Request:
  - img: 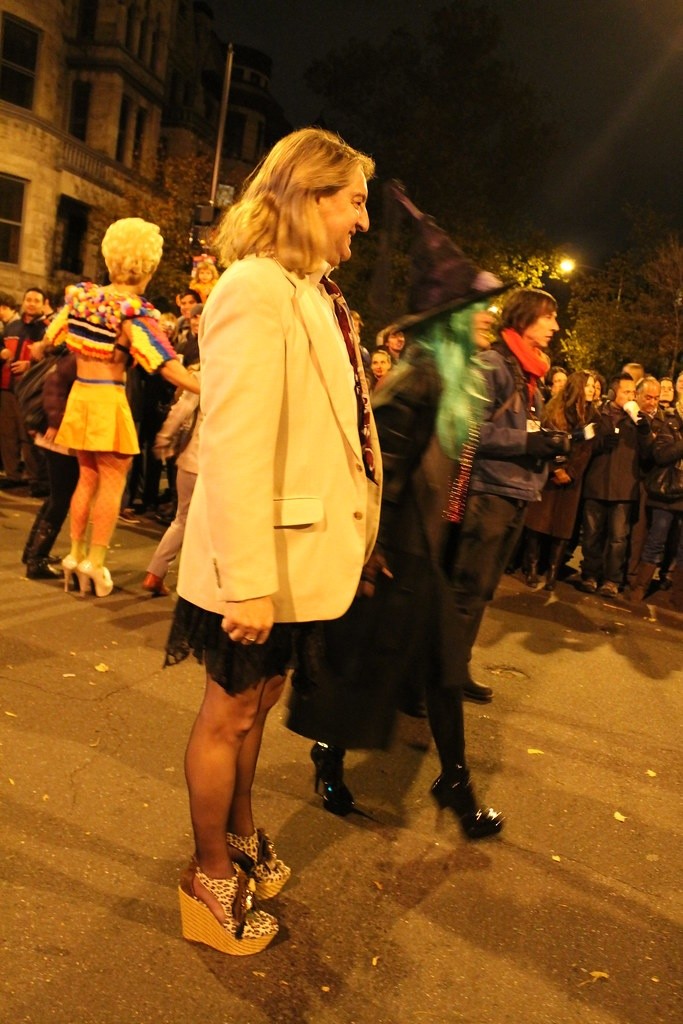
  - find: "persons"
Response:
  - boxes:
[449,288,560,698]
[27,217,201,597]
[21,355,79,580]
[192,262,217,295]
[0,287,65,497]
[505,363,683,608]
[142,370,204,595]
[283,300,502,840]
[120,289,205,525]
[160,129,383,956]
[350,310,405,390]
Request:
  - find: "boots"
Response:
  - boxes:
[624,562,656,601]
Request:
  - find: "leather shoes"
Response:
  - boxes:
[21,549,61,564]
[27,560,63,580]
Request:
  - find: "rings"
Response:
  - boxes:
[244,636,255,643]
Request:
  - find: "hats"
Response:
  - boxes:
[367,180,517,335]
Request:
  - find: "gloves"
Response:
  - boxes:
[525,431,567,461]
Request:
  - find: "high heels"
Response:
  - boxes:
[224,827,291,901]
[311,741,354,816]
[177,867,278,955]
[76,560,113,597]
[431,772,503,839]
[61,554,79,592]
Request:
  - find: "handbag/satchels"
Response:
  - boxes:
[643,428,683,501]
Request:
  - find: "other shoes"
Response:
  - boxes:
[397,695,426,716]
[463,676,493,702]
[142,573,170,595]
[118,508,141,523]
[29,485,48,497]
[507,555,620,597]
